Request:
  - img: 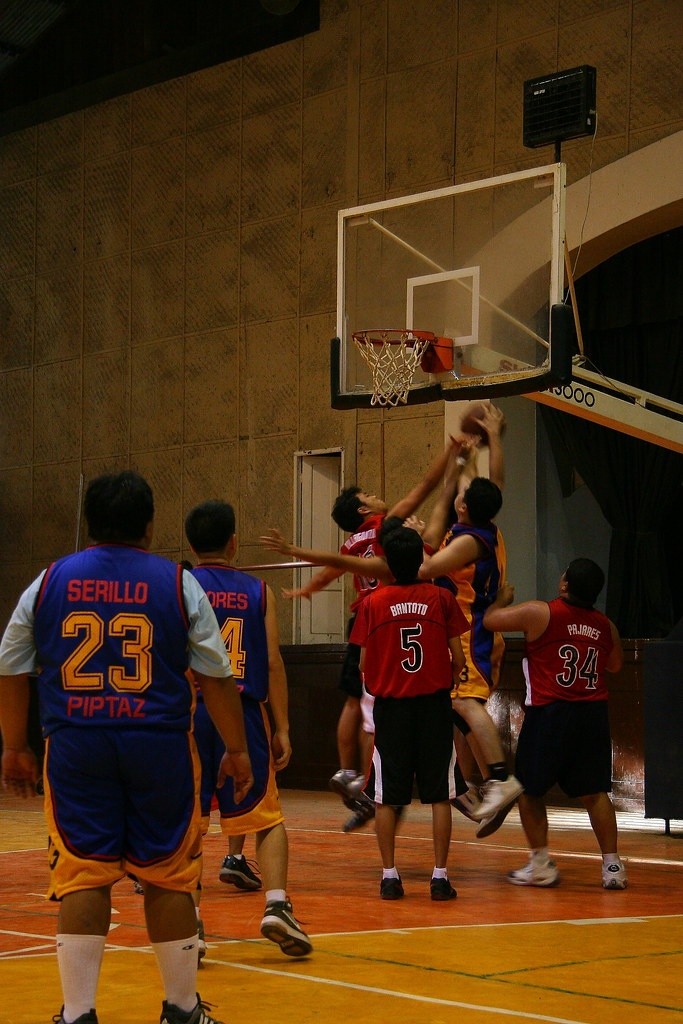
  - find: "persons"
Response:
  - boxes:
[348,527,472,901]
[260,402,526,839]
[148,501,313,963]
[0,471,254,1023]
[483,558,629,889]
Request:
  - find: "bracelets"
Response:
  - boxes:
[456,456,467,468]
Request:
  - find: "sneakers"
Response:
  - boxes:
[346,774,371,801]
[134,881,145,895]
[473,774,525,819]
[260,896,313,956]
[52,1004,98,1024]
[380,873,404,900]
[159,992,224,1024]
[430,878,456,900]
[474,799,516,838]
[219,854,262,889]
[342,806,375,833]
[197,919,206,964]
[329,768,358,802]
[505,860,561,888]
[601,856,629,888]
[450,780,483,824]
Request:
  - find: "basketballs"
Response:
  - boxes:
[461,401,506,440]
